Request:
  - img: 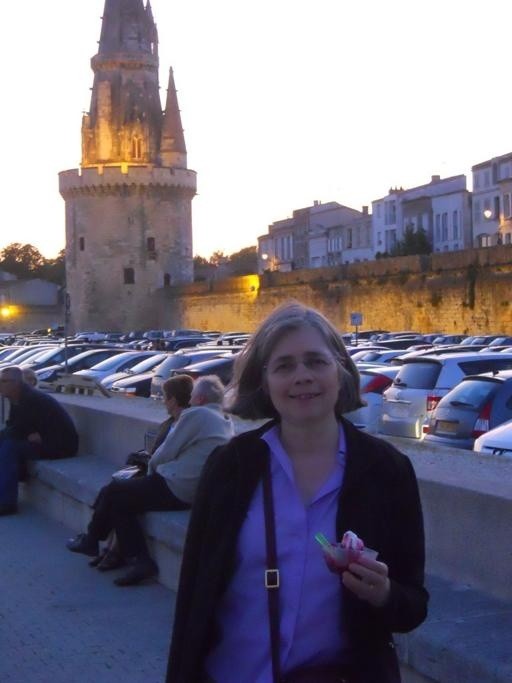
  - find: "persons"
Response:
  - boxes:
[89,374,195,571]
[0,367,79,515]
[66,374,235,586]
[165,302,430,683]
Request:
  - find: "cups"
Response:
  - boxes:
[327,544,378,574]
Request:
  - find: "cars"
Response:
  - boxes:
[0,327,252,399]
[341,329,512,457]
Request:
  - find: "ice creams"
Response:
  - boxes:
[318,531,365,573]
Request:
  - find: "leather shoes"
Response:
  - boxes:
[64,528,160,587]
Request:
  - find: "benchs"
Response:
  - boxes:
[19,450,509,681]
